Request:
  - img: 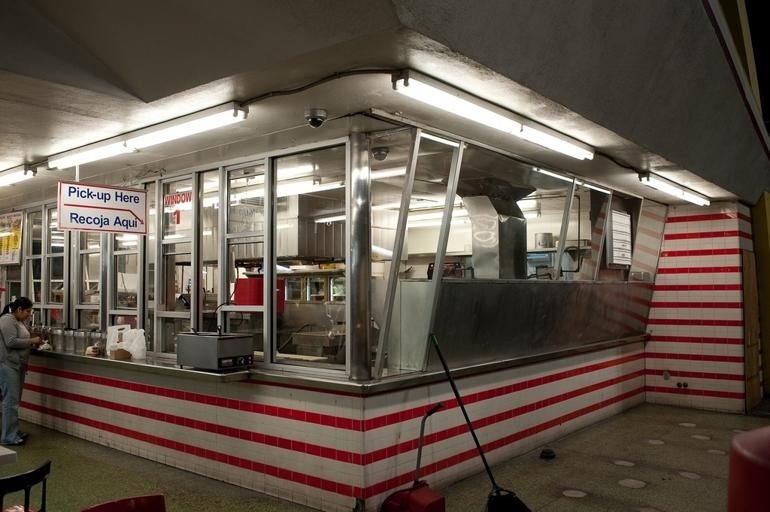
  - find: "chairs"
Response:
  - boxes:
[81,494,167,512]
[0,457,52,512]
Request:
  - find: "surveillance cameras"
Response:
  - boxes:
[304,109,327,128]
[372,147,389,160]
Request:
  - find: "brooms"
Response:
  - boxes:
[430,332,531,511]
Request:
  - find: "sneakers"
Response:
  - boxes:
[0,430,29,445]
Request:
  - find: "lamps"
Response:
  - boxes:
[391,68,595,162]
[637,170,710,207]
[44,101,249,170]
[2,166,37,186]
[166,157,410,206]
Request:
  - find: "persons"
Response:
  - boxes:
[0,296,45,446]
